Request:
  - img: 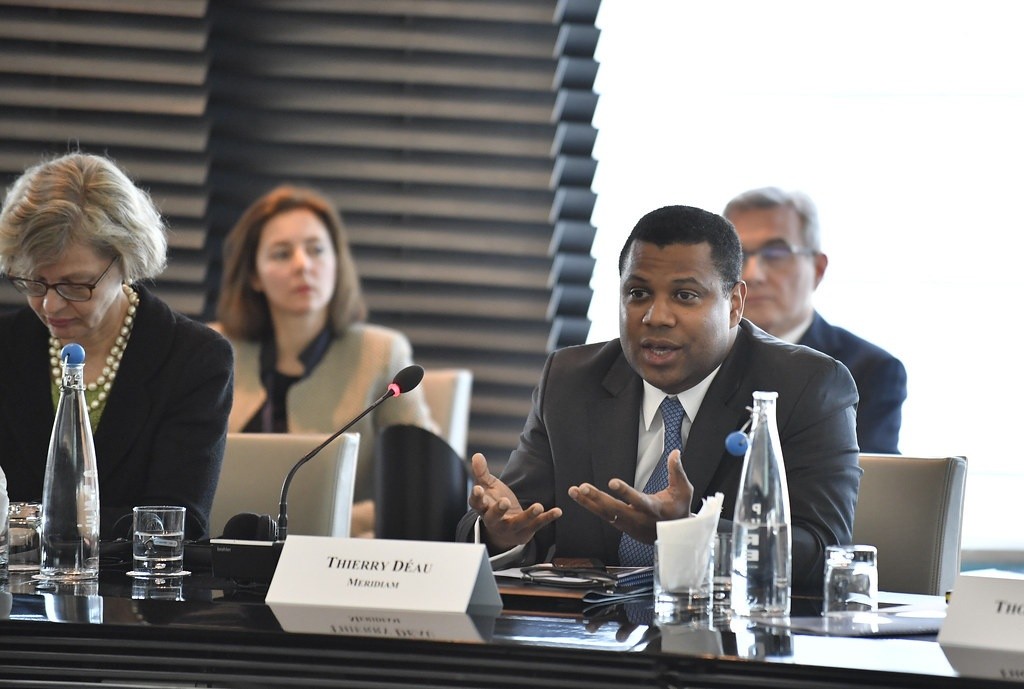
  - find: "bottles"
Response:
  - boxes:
[1,466,10,567]
[40,344,101,579]
[730,390,792,617]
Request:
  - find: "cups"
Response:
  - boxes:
[8,500,41,573]
[711,532,749,607]
[651,538,715,613]
[132,506,186,574]
[822,545,879,626]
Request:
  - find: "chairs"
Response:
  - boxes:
[852,453,968,597]
[208,432,361,540]
[420,367,473,460]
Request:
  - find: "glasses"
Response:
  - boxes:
[740,244,815,269]
[1,256,120,302]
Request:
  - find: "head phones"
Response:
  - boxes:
[192,513,278,546]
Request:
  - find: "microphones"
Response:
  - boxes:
[209,366,425,583]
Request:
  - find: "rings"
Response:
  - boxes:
[608,514,617,524]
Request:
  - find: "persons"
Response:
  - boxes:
[454,206,864,593]
[0,153,236,542]
[200,186,441,538]
[721,187,907,455]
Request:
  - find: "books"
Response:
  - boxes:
[491,561,655,588]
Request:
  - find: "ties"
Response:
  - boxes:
[618,399,686,568]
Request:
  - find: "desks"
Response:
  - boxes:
[0,538,1024,689]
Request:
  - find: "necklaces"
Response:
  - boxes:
[48,284,140,413]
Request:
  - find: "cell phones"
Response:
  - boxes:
[553,556,607,576]
